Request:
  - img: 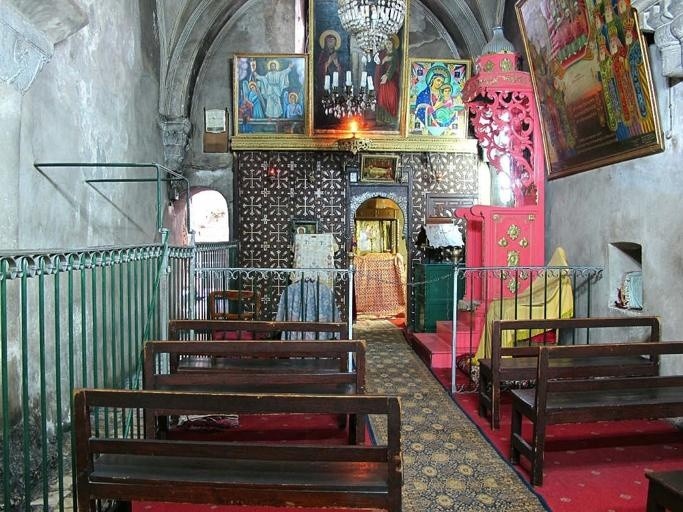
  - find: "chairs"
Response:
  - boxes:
[208,289,263,340]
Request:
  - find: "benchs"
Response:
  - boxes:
[477,316,683,488]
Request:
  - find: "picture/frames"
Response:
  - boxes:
[360,153,401,185]
[353,216,397,257]
[309,0,410,139]
[232,53,309,139]
[408,57,472,142]
[291,219,318,251]
[513,0,664,182]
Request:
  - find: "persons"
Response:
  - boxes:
[315,28,345,103]
[414,63,451,126]
[434,82,452,126]
[285,91,303,119]
[372,32,400,116]
[523,1,655,159]
[250,58,294,122]
[244,81,264,118]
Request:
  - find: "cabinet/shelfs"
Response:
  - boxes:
[413,263,465,332]
